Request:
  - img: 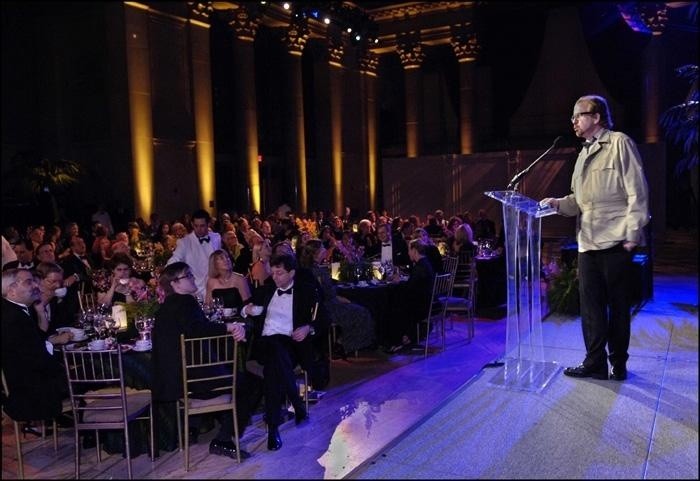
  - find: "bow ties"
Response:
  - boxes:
[79,255,87,260]
[21,261,31,267]
[383,243,391,247]
[22,306,32,315]
[199,234,210,244]
[582,135,597,148]
[277,286,294,296]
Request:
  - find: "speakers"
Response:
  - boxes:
[632,253,653,306]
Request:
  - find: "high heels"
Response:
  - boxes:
[20,423,54,439]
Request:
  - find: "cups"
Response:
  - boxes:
[120,279,129,285]
[136,340,149,348]
[354,267,364,288]
[251,305,264,316]
[366,268,372,288]
[89,340,103,350]
[55,287,66,297]
[72,329,86,341]
[223,308,235,317]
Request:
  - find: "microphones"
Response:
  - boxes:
[507,135,565,189]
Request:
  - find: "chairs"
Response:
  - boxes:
[176,333,241,472]
[1,369,81,480]
[266,302,319,433]
[62,343,155,480]
[417,272,454,358]
[443,252,459,296]
[436,257,475,344]
[449,246,478,317]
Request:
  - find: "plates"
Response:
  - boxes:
[133,346,153,351]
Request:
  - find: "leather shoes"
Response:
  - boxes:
[563,363,609,380]
[294,407,311,429]
[610,365,627,380]
[209,440,250,459]
[267,425,283,452]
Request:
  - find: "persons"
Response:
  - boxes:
[63,235,94,274]
[0,268,104,449]
[366,224,409,267]
[537,95,649,382]
[412,227,444,275]
[46,225,70,260]
[381,207,393,223]
[263,241,325,305]
[153,262,259,462]
[29,228,44,249]
[272,209,317,241]
[379,215,388,224]
[62,222,79,247]
[383,238,436,354]
[237,253,328,450]
[92,221,189,266]
[440,216,462,242]
[303,239,372,361]
[3,238,41,270]
[31,261,64,340]
[463,211,476,229]
[35,243,90,295]
[451,223,480,296]
[391,209,446,238]
[95,252,147,313]
[476,209,496,238]
[223,210,271,260]
[164,209,222,298]
[317,207,378,259]
[205,249,253,311]
[494,221,505,262]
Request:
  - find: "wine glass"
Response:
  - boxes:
[212,296,224,324]
[134,316,150,349]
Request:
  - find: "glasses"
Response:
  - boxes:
[570,111,596,122]
[174,273,193,282]
